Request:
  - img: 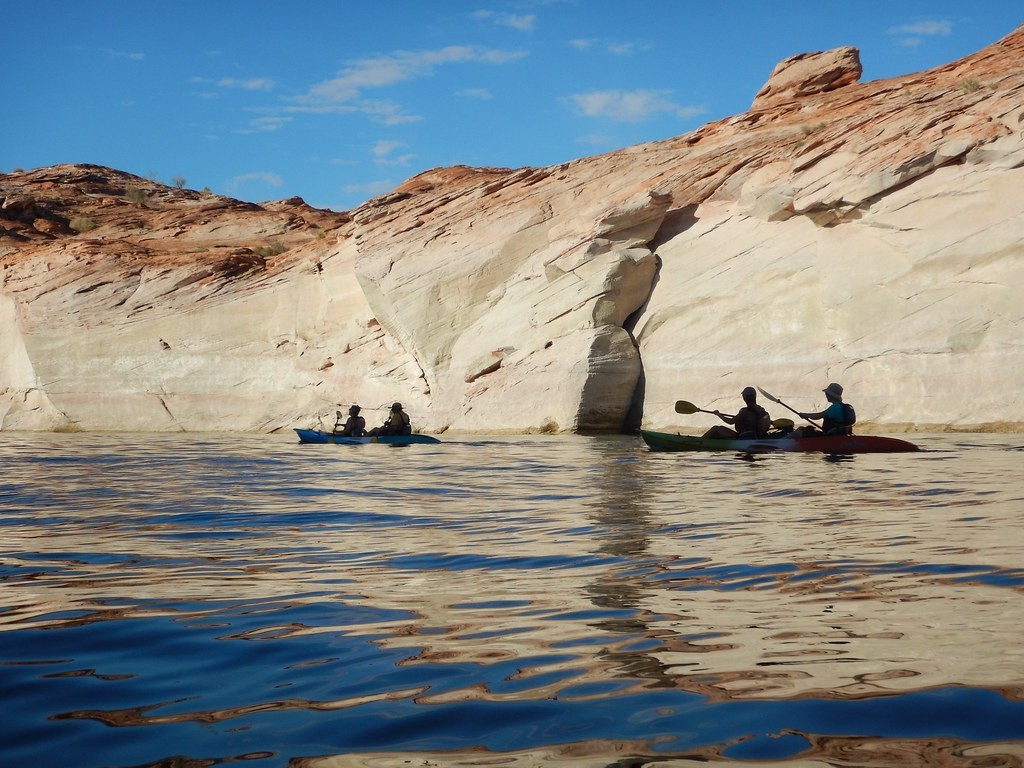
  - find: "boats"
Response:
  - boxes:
[293,428,443,444]
[635,427,919,453]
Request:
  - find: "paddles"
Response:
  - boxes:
[370,410,394,443]
[756,385,822,430]
[327,410,342,444]
[675,400,794,429]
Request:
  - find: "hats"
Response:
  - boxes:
[388,403,405,409]
[823,383,843,401]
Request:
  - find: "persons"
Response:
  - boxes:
[364,403,411,436]
[326,405,366,437]
[785,383,853,437]
[702,387,771,438]
